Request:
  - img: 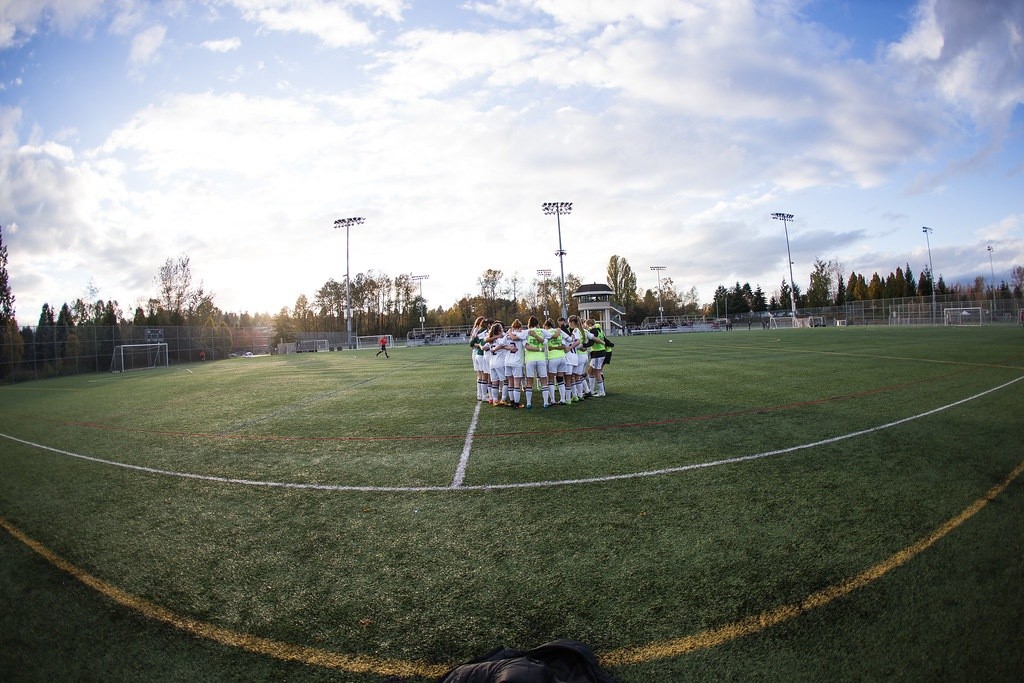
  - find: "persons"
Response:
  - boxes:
[376,335,390,358]
[659,319,693,329]
[469,314,614,409]
[1018,311,1024,327]
[747,320,751,330]
[622,325,640,336]
[762,320,769,329]
[200,350,205,363]
[409,330,471,339]
[728,322,732,331]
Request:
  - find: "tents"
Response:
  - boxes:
[961,311,970,316]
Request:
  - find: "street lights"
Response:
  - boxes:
[412,275,429,332]
[537,269,552,321]
[923,226,936,324]
[987,246,996,310]
[333,216,366,345]
[771,213,796,317]
[650,267,666,321]
[541,202,573,323]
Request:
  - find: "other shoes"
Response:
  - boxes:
[488,400,494,404]
[558,401,567,406]
[376,354,379,357]
[566,399,572,406]
[482,398,489,403]
[513,402,525,409]
[387,355,390,358]
[578,395,585,401]
[477,397,482,401]
[527,405,534,409]
[542,403,551,408]
[572,396,580,403]
[507,399,514,407]
[492,400,501,407]
[549,402,558,406]
[500,399,508,406]
[583,392,594,400]
[592,392,606,398]
